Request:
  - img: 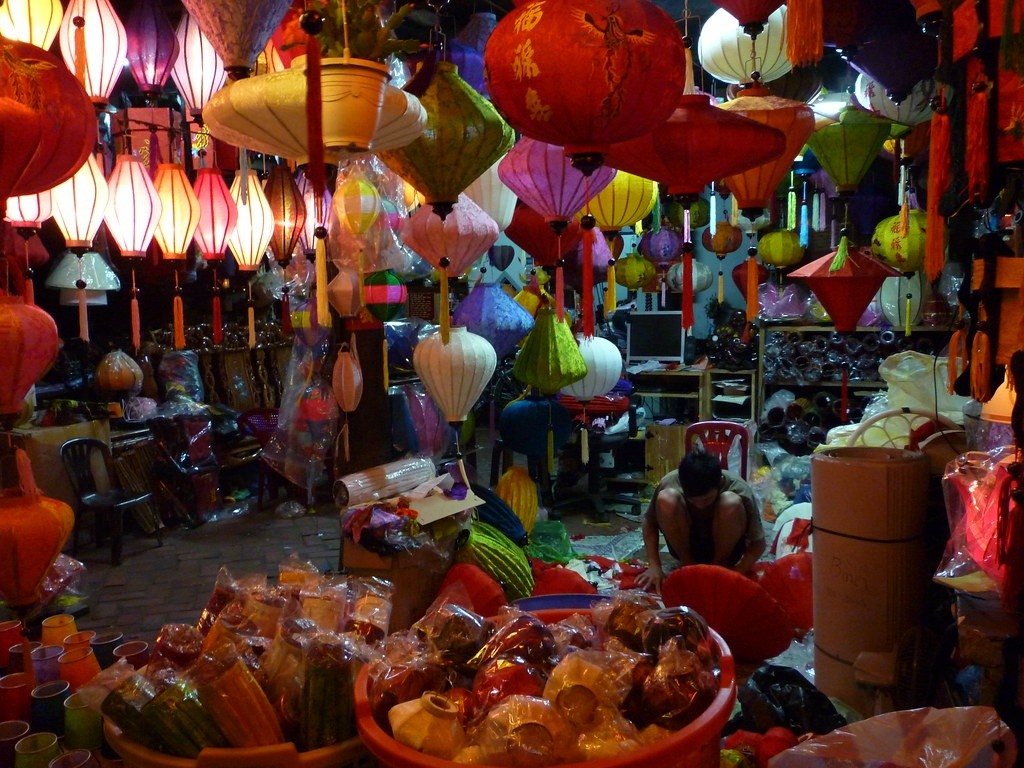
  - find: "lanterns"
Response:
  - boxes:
[0,0,1024,767]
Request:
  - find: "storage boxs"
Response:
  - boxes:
[339,534,440,636]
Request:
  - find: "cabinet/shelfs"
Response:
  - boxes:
[15,417,114,554]
[636,366,756,423]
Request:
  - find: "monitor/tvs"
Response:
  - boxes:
[626,310,685,365]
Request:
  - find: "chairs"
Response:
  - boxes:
[685,420,749,483]
[59,438,164,564]
[236,407,338,509]
